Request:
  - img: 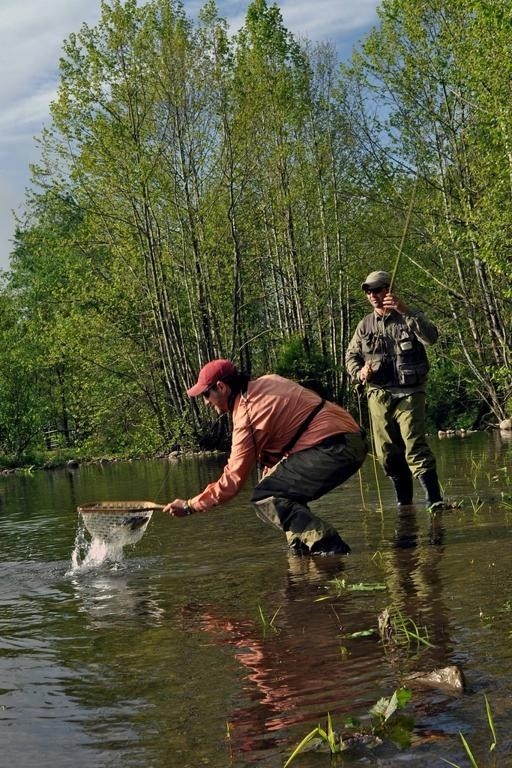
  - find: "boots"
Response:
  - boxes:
[312,537,351,556]
[388,474,415,506]
[417,469,444,508]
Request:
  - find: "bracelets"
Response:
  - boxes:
[187,499,194,511]
[183,501,192,515]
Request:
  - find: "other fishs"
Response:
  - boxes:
[123,515,149,532]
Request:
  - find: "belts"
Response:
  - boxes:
[322,426,368,445]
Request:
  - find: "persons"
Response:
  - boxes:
[343,269,445,510]
[378,508,454,746]
[160,359,370,555]
[175,595,383,751]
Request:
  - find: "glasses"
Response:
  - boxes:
[203,387,217,399]
[365,288,383,296]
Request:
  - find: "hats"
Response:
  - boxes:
[361,271,393,290]
[186,358,236,397]
